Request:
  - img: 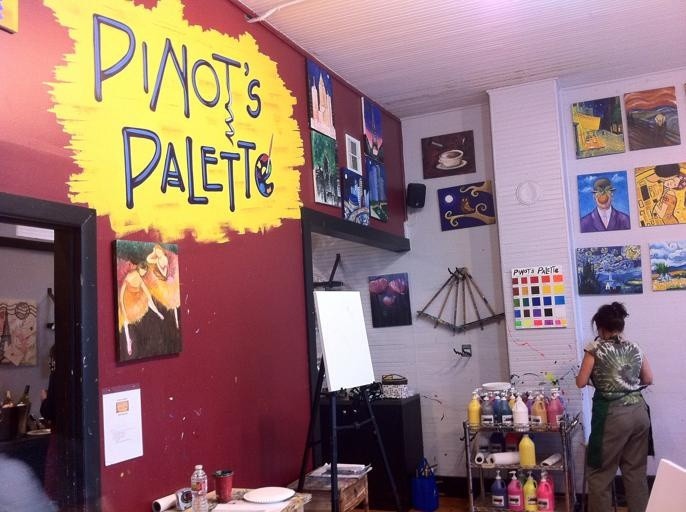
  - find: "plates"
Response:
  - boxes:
[243,485,296,504]
[436,160,467,171]
[27,428,51,435]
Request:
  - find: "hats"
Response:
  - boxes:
[590,178,617,194]
[645,164,680,184]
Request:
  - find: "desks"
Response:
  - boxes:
[162,486,313,511]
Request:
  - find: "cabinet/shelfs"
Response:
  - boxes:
[288,466,369,512]
[462,412,578,512]
[319,393,423,512]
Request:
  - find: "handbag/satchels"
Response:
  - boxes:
[411,456,439,512]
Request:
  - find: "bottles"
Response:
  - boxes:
[1,383,30,408]
[190,464,209,512]
[477,431,536,468]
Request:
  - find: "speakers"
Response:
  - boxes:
[407,183,426,208]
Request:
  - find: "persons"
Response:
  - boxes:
[39,344,57,503]
[576,300,655,512]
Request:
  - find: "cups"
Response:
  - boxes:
[438,149,464,167]
[212,469,234,503]
